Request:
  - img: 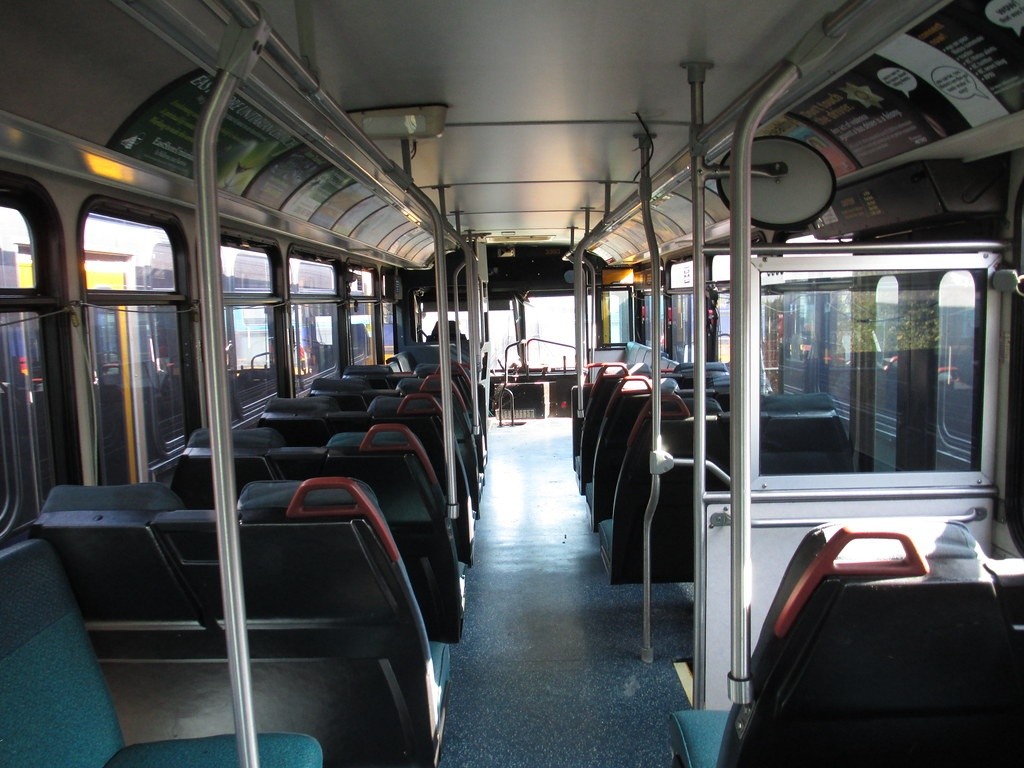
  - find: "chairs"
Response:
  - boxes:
[2,535,323,768]
[670,512,1024,768]
[571,340,859,584]
[30,336,486,762]
